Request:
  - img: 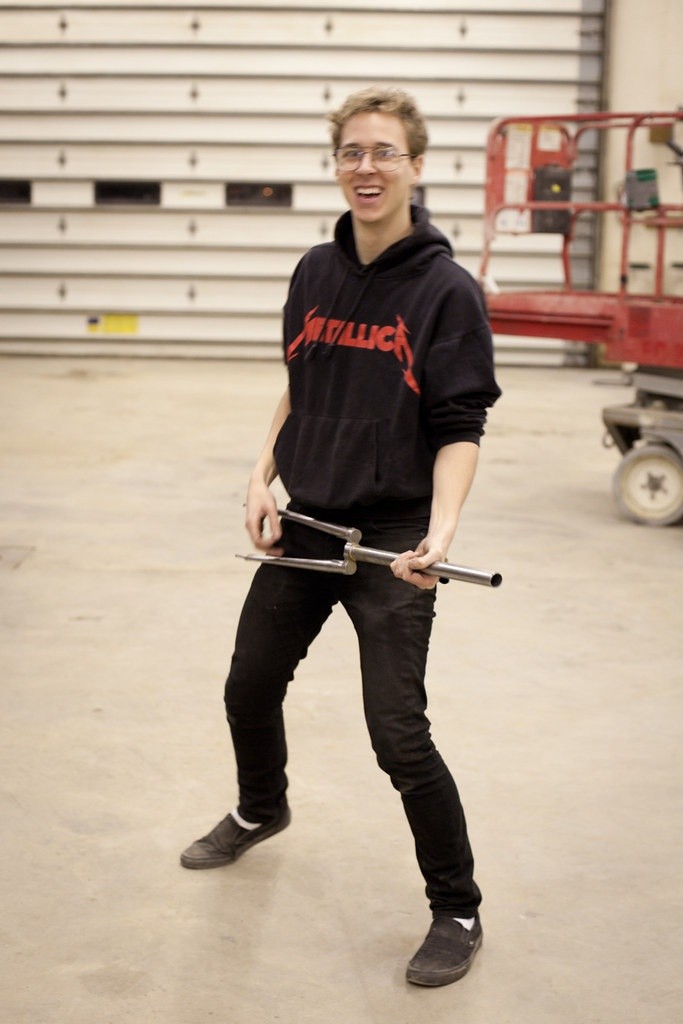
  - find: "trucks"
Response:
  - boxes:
[469,112,683,534]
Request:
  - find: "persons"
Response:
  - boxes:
[178,86,503,988]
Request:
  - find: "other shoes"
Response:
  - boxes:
[406,913,484,986]
[180,804,291,869]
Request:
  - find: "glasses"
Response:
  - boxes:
[333,146,417,173]
